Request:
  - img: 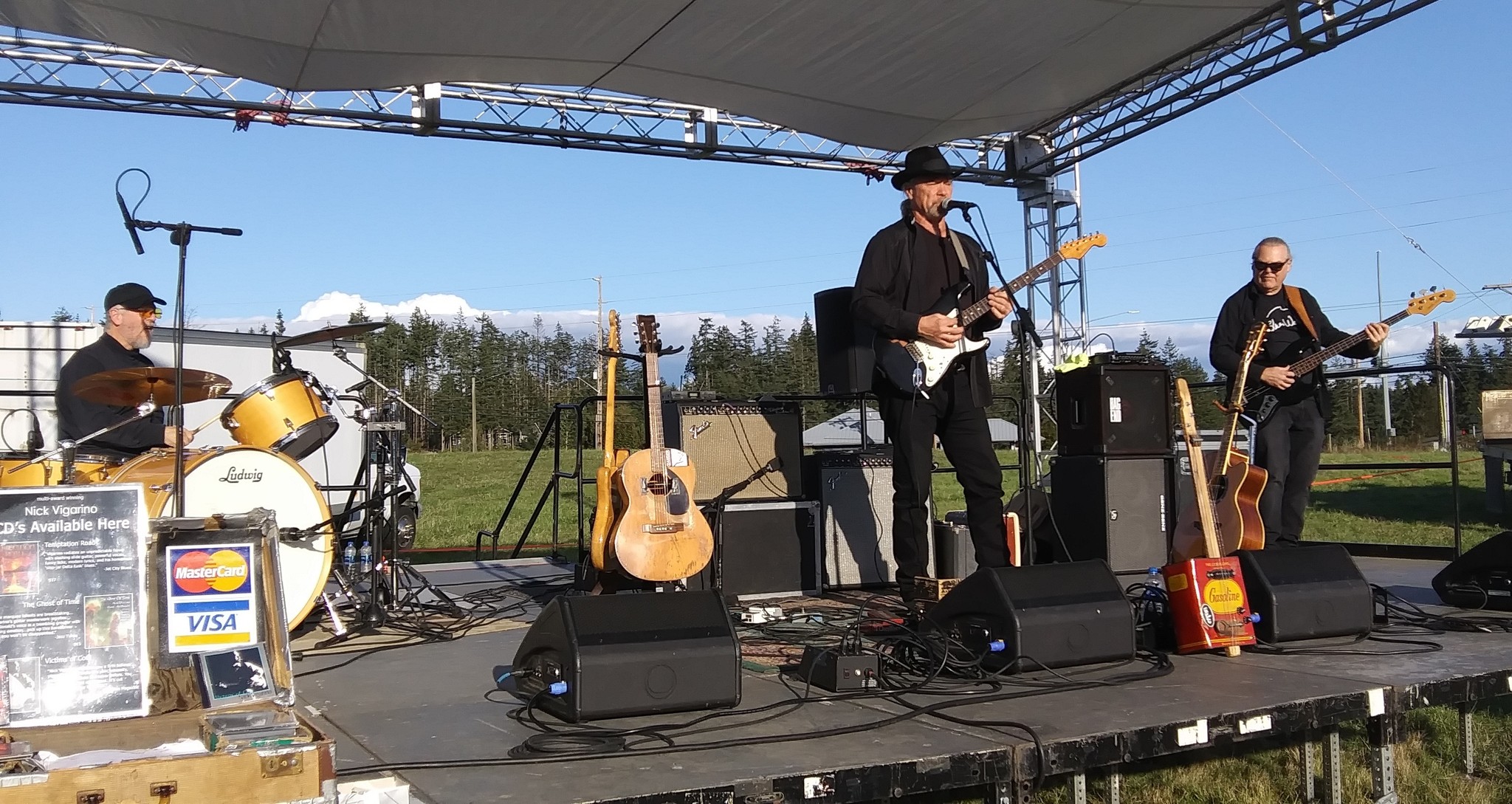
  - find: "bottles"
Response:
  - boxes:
[1144,568,1165,611]
[345,541,355,579]
[359,542,372,573]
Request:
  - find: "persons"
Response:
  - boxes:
[1210,237,1390,544]
[852,147,1017,602]
[55,282,195,457]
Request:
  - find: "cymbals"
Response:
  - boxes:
[273,320,392,347]
[71,367,233,405]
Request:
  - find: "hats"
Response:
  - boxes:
[104,283,167,308]
[891,144,966,191]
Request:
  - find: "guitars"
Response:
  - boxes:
[871,231,1107,394]
[590,309,714,583]
[1171,319,1269,567]
[1226,286,1457,432]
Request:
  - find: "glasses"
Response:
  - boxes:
[115,307,163,319]
[1253,259,1290,272]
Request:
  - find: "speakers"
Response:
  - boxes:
[512,588,742,724]
[1227,543,1373,643]
[804,451,938,590]
[1049,455,1176,576]
[662,399,806,505]
[1432,530,1512,612]
[1054,363,1173,456]
[915,558,1135,672]
[813,286,877,396]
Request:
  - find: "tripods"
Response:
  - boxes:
[313,341,465,650]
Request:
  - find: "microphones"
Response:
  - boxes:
[757,456,784,473]
[116,191,144,255]
[34,417,44,449]
[345,376,380,393]
[942,198,976,210]
[272,333,281,374]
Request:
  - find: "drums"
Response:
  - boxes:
[100,444,333,633]
[219,369,340,464]
[0,451,129,488]
